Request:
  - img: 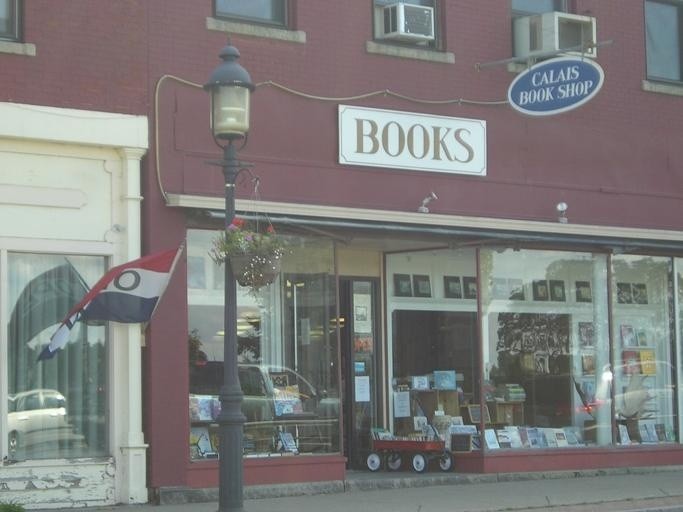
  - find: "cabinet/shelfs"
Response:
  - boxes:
[488,398,525,427]
[416,392,459,424]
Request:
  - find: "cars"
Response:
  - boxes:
[5,388,105,459]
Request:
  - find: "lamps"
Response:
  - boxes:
[556,199,569,224]
[417,188,439,213]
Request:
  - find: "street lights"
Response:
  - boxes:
[201,35,260,512]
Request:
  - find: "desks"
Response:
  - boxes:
[245,419,337,454]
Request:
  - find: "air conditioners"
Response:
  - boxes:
[515,15,596,65]
[382,2,435,45]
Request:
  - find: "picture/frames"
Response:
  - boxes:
[393,273,648,305]
[576,320,658,406]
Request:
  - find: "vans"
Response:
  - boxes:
[543,357,683,447]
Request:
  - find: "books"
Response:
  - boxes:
[393,273,665,449]
[189,385,303,456]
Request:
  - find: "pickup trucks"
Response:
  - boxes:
[188,360,340,446]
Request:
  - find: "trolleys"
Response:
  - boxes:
[367,396,456,474]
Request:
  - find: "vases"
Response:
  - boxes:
[232,255,281,286]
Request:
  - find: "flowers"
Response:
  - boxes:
[211,216,293,300]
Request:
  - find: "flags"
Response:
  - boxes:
[36,248,184,362]
